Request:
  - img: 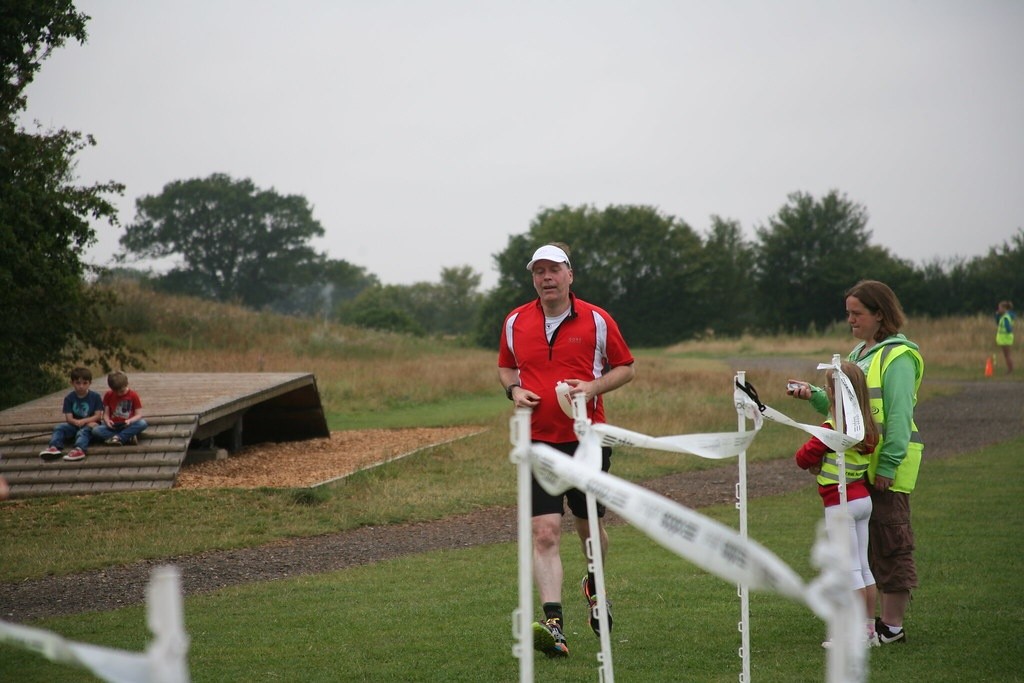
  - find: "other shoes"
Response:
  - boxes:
[822,636,872,650]
[104,437,123,447]
[869,632,883,649]
[129,434,138,445]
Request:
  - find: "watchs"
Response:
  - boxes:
[506,384,520,400]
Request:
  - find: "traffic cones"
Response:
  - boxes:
[984,358,993,376]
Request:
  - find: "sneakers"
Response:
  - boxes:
[873,616,907,646]
[63,449,86,461]
[39,447,63,459]
[581,574,614,636]
[530,619,570,661]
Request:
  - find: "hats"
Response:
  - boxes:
[525,244,571,269]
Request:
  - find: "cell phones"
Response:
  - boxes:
[786,384,817,393]
[113,422,126,427]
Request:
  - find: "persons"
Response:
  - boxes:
[994,301,1016,374]
[499,242,635,658]
[786,281,923,644]
[797,361,880,650]
[39,367,104,461]
[92,372,148,446]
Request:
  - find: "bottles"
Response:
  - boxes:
[555,380,577,419]
